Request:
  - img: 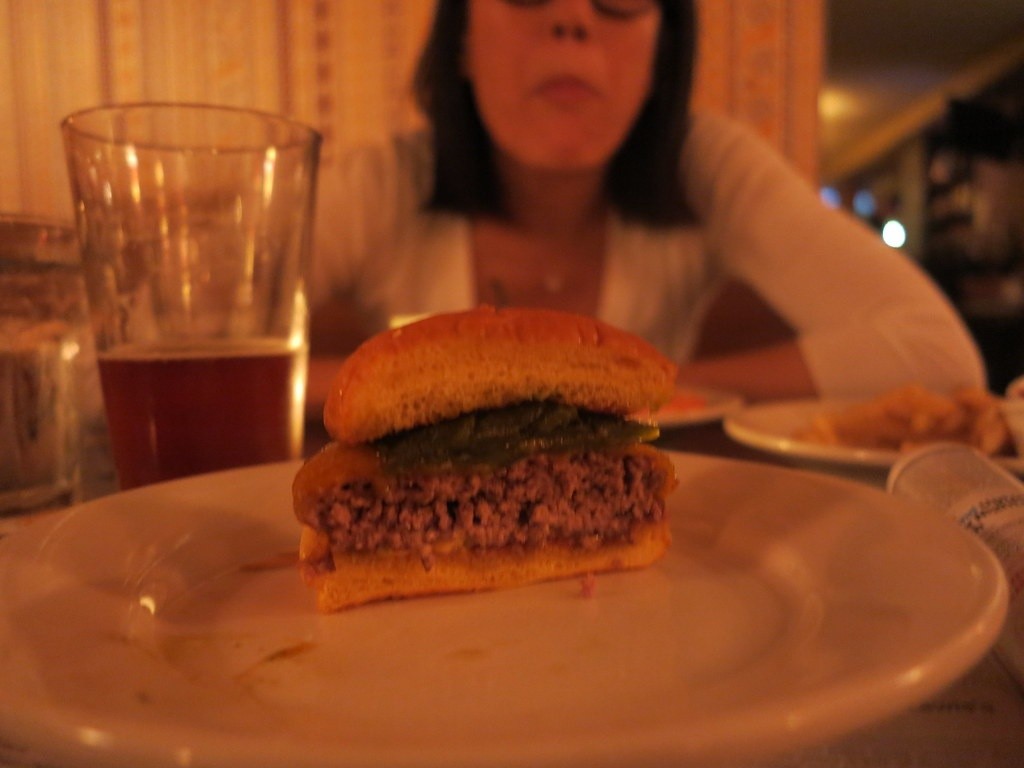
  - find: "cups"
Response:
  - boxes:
[0,214,86,517]
[59,101,317,494]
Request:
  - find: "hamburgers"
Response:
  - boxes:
[290,302,679,612]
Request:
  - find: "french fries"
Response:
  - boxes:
[809,374,1011,462]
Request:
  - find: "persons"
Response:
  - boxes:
[294,0,981,447]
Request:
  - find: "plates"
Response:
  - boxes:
[305,355,749,428]
[723,389,1024,476]
[0,447,1009,768]
